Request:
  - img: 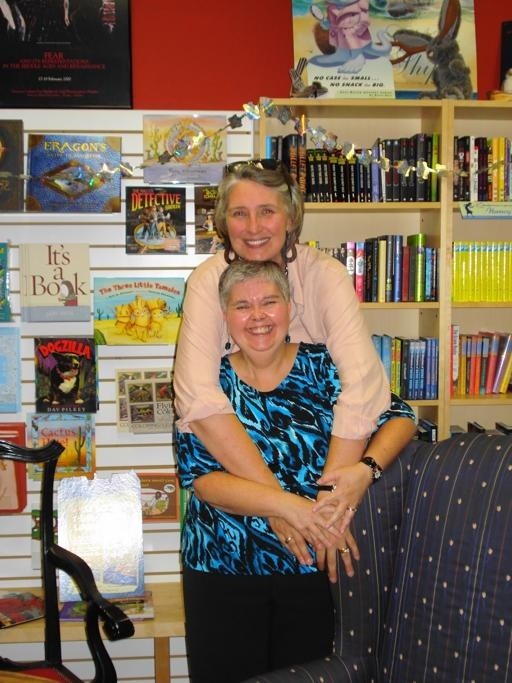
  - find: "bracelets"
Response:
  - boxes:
[317,482,336,492]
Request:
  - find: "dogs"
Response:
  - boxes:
[40,350,86,407]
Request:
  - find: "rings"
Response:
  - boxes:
[285,536,292,543]
[347,507,357,512]
[340,547,349,553]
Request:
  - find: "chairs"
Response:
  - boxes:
[2,438,139,683]
[243,430,511,683]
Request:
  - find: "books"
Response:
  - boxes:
[373,333,437,400]
[454,136,512,202]
[452,420,511,438]
[453,240,512,303]
[265,131,439,201]
[450,324,512,398]
[411,418,437,443]
[302,233,439,302]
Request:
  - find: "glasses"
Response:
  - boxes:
[226,157,292,206]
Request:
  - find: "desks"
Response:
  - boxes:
[1,582,194,683]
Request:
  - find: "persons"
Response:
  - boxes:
[175,259,419,683]
[170,154,390,585]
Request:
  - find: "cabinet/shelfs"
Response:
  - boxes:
[254,94,509,439]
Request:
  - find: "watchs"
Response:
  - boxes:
[361,457,383,480]
